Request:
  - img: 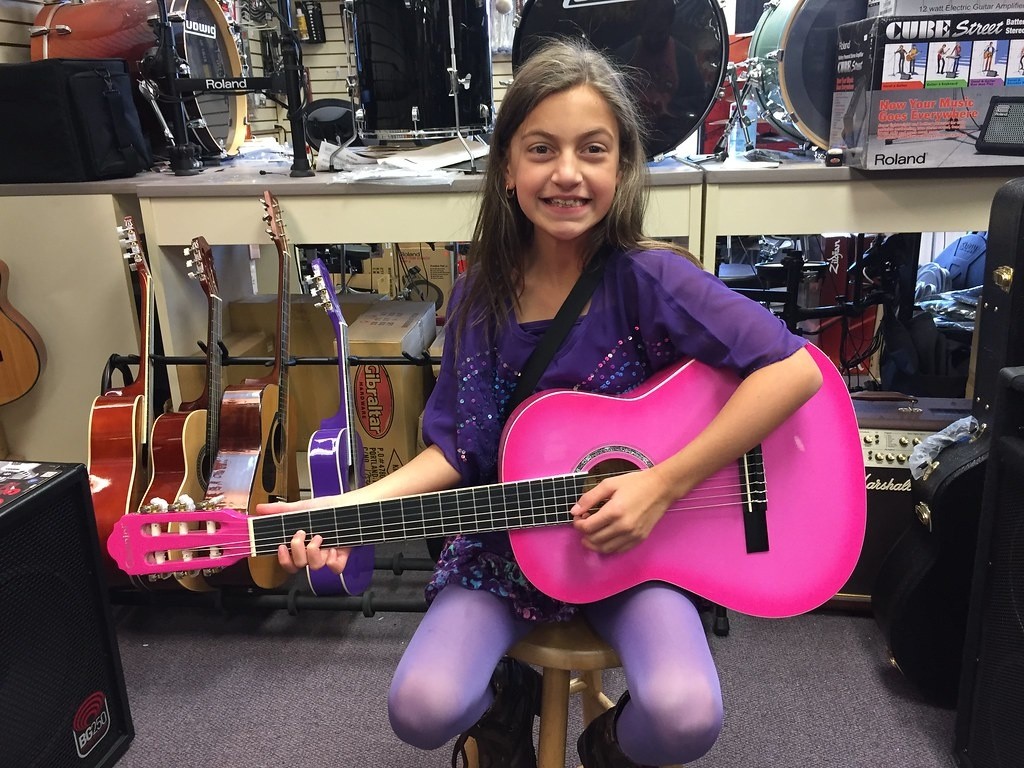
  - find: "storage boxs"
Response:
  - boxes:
[329,242,454,325]
[229,294,389,452]
[177,331,274,402]
[334,300,437,486]
[828,12,1024,170]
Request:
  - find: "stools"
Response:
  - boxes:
[460,620,622,768]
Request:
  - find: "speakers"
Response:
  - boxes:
[953,367,1024,768]
[0,460,136,768]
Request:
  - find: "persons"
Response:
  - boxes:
[256,43,824,768]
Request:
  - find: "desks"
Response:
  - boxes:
[0,166,222,469]
[686,150,1024,275]
[135,156,702,412]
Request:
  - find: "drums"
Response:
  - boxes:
[30,0,252,162]
[745,0,866,157]
[346,1,496,149]
[511,0,731,165]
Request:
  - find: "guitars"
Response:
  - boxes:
[135,231,224,597]
[305,257,383,598]
[202,186,290,601]
[0,258,48,408]
[107,338,868,619]
[88,214,155,559]
